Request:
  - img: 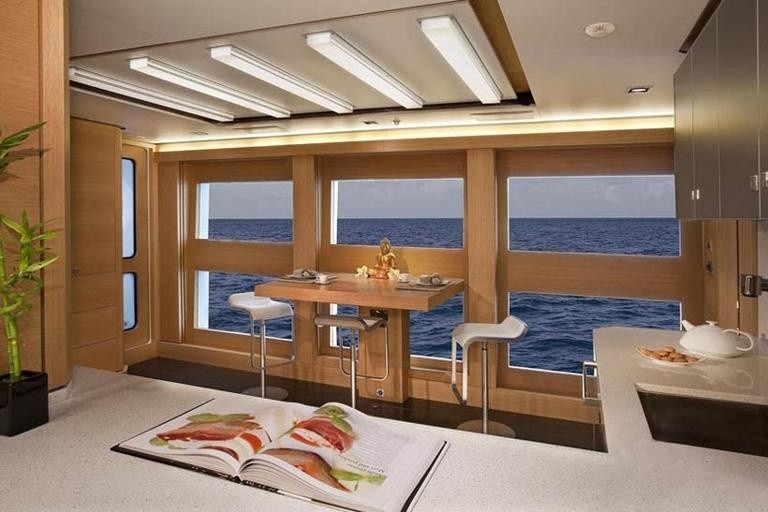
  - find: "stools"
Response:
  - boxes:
[228,291,298,400]
[451,315,529,438]
[314,314,389,410]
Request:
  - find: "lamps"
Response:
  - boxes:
[130,58,292,119]
[211,46,354,114]
[69,68,234,122]
[416,15,503,105]
[306,32,423,109]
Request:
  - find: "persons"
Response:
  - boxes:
[367,237,397,279]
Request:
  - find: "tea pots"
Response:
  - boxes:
[704,360,756,391]
[679,313,755,361]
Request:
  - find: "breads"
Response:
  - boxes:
[642,345,700,364]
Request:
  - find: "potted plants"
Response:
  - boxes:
[0,121,62,436]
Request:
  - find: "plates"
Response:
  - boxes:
[316,281,330,284]
[278,276,336,284]
[417,279,449,286]
[632,345,707,364]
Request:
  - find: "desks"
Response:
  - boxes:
[255,271,465,404]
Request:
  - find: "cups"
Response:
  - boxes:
[317,275,328,282]
[399,274,409,282]
[419,276,428,283]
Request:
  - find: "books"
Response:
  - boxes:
[108,393,451,512]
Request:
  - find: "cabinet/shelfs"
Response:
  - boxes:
[674,12,722,219]
[718,0,768,219]
